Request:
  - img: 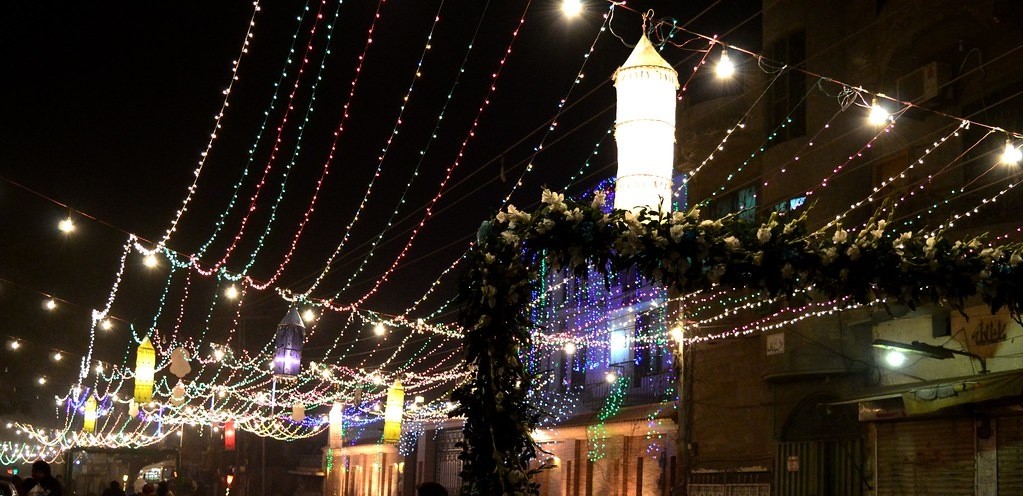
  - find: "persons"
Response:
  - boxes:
[101,479,174,496]
[417,482,449,496]
[14,461,66,496]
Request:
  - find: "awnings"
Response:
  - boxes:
[817,368,1023,490]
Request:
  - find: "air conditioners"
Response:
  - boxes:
[896,62,956,122]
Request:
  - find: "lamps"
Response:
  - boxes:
[224,283,237,298]
[871,339,989,375]
[143,250,160,270]
[227,472,234,484]
[612,14,678,229]
[271,304,317,375]
[1001,136,1019,165]
[867,98,889,124]
[329,320,405,449]
[58,214,77,235]
[714,50,735,79]
[8,298,236,452]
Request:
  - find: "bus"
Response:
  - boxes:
[134,458,189,494]
